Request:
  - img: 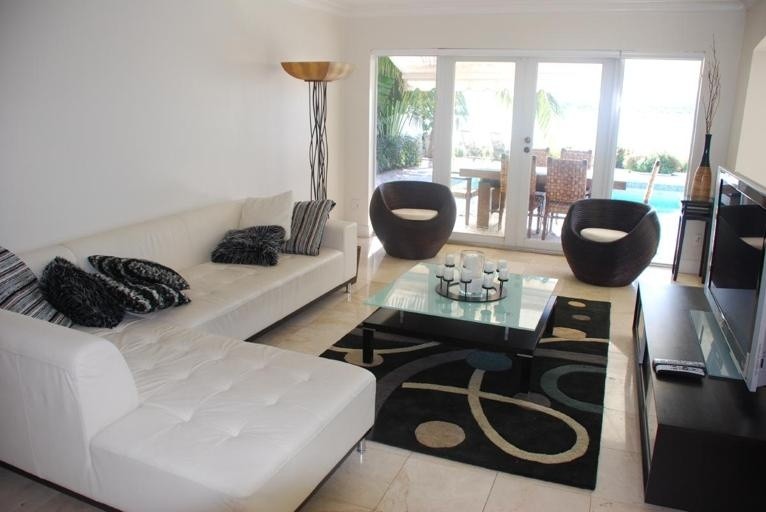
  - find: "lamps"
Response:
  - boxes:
[281,60,350,200]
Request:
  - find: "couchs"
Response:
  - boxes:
[0,198,380,512]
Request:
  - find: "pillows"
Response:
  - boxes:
[237,189,294,241]
[0,246,192,331]
[280,200,338,257]
[211,225,284,269]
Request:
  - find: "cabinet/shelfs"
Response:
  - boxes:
[632,282,766,511]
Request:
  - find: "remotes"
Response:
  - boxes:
[652,359,708,375]
[656,365,706,381]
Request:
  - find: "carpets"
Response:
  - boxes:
[318,295,611,491]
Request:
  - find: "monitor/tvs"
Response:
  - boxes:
[686,166,766,394]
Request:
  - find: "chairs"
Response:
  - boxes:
[711,206,765,290]
[368,180,457,261]
[450,146,662,242]
[560,197,661,289]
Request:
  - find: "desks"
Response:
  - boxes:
[673,199,714,284]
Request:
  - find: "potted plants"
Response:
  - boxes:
[689,34,722,203]
